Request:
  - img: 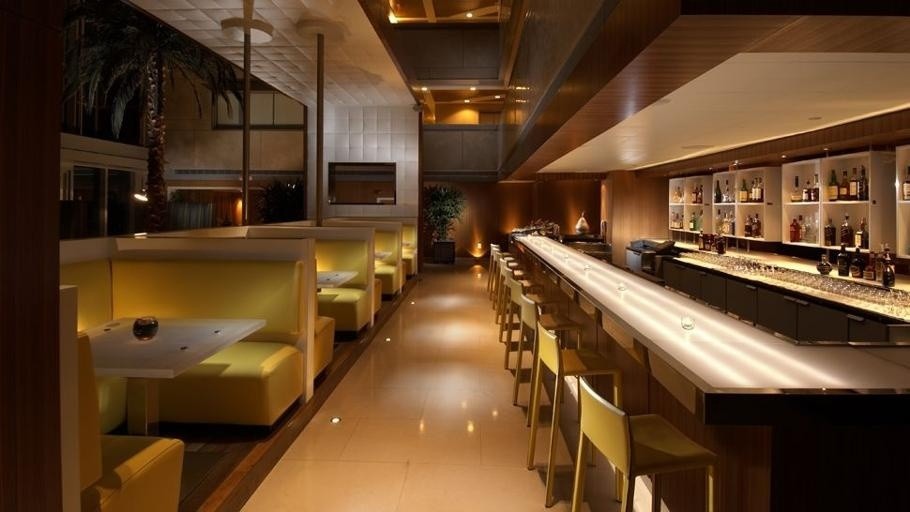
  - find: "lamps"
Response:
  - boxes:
[133,185,148,203]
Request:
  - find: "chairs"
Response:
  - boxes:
[571,372,721,511]
[485,231,632,424]
[524,323,624,510]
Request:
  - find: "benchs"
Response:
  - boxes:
[314,239,371,333]
[313,259,335,385]
[374,233,383,316]
[76,329,184,512]
[382,231,400,297]
[110,256,306,445]
[403,225,417,277]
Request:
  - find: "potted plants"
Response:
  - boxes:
[423,182,466,265]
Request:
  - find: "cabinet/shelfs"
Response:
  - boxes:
[662,261,910,343]
[712,167,781,243]
[780,151,894,254]
[668,175,710,236]
[895,144,910,258]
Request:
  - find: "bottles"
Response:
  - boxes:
[673,187,684,203]
[715,180,734,202]
[739,178,764,202]
[902,166,910,200]
[692,183,703,203]
[671,211,683,229]
[791,174,819,202]
[745,213,762,237]
[790,213,818,243]
[688,209,702,231]
[817,243,896,287]
[825,216,868,249]
[715,208,734,236]
[827,168,868,201]
[698,231,726,254]
[133,316,158,341]
